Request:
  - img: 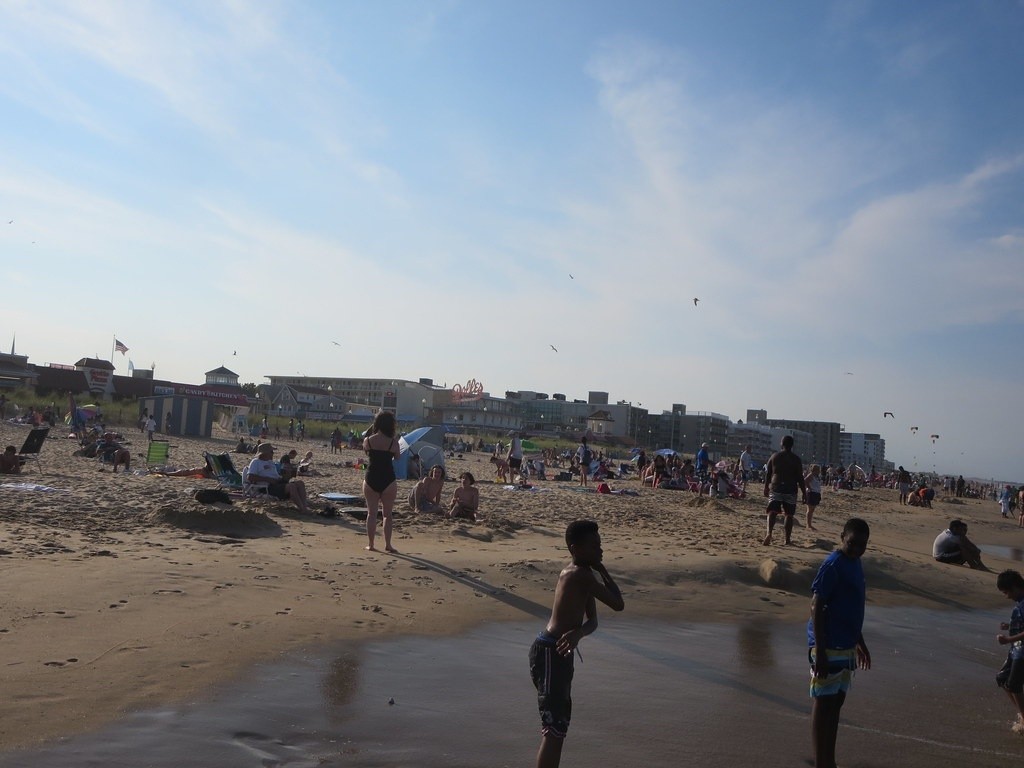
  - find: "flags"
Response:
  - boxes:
[116,340,123,347]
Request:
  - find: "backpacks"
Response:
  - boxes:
[577,446,591,465]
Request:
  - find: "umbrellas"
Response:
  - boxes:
[69,395,81,433]
[392,427,433,457]
[65,404,97,424]
[653,448,681,459]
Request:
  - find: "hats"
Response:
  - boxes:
[506,430,514,436]
[258,443,277,452]
[702,443,709,447]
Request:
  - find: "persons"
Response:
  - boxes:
[236,437,318,515]
[261,416,305,442]
[696,442,715,497]
[807,518,872,768]
[890,466,942,509]
[944,474,997,500]
[637,451,695,489]
[154,464,218,480]
[0,446,19,474]
[164,412,172,436]
[599,462,618,479]
[0,394,55,427]
[363,412,400,552]
[407,454,420,479]
[763,435,822,546]
[138,408,148,433]
[506,430,524,485]
[490,439,509,484]
[478,439,484,452]
[445,472,479,522]
[738,444,751,494]
[804,460,877,490]
[96,423,132,474]
[998,485,1024,528]
[146,415,156,440]
[996,569,1024,735]
[572,437,591,487]
[330,427,341,454]
[528,519,624,768]
[409,464,445,514]
[933,519,991,572]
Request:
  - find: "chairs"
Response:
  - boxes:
[15,426,51,477]
[242,465,290,513]
[92,439,126,473]
[137,439,170,478]
[202,451,244,492]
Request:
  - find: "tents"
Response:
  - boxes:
[393,441,444,480]
[505,439,542,456]
[586,461,604,474]
[631,454,652,463]
